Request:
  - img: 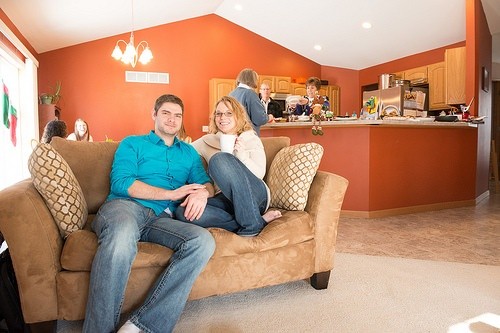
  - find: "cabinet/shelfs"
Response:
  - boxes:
[208,75,339,122]
[395,46,466,111]
[38,103,61,138]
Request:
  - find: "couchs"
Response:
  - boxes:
[0,140,350,333]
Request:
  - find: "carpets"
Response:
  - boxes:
[56,252,500,333]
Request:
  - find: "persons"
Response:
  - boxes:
[82,95,216,333]
[257,84,282,118]
[68,119,93,142]
[177,95,283,236]
[230,69,273,135]
[289,77,329,116]
[41,120,67,144]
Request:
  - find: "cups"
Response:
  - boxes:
[219,134,239,154]
[106,138,113,142]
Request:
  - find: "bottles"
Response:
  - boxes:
[352,111,357,118]
[344,112,350,118]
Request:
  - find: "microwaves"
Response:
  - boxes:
[258,92,291,115]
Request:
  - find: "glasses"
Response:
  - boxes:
[214,111,232,118]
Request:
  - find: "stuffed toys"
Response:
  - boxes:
[309,96,325,135]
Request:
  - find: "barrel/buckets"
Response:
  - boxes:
[393,79,410,90]
[376,72,395,90]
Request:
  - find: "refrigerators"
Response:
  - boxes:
[362,87,430,119]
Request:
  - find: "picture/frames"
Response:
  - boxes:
[483,66,489,93]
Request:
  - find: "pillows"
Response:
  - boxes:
[266,142,324,212]
[28,143,88,240]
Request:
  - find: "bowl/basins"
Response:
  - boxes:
[298,116,310,122]
[274,119,287,123]
[430,116,457,122]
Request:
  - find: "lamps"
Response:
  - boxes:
[111,1,153,68]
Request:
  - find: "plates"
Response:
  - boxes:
[333,117,357,121]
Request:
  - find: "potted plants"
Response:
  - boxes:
[41,81,61,105]
